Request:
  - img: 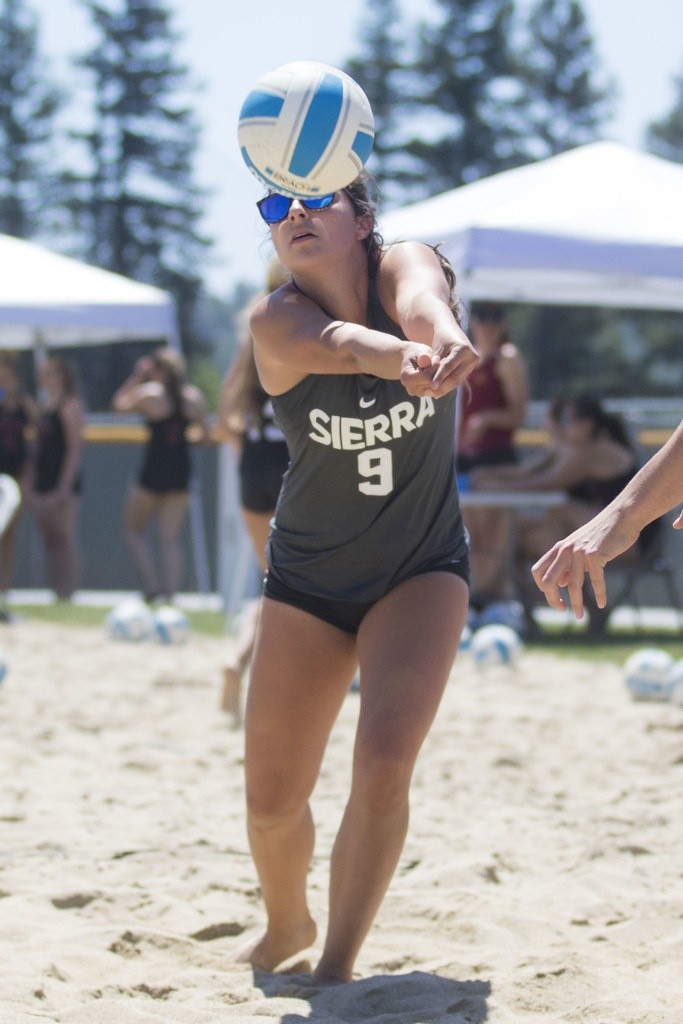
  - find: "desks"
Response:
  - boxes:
[450,490,567,625]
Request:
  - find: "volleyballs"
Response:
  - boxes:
[236,60,377,203]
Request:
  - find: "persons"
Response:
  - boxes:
[20,362,85,606]
[514,395,655,566]
[530,420,681,620]
[245,177,484,990]
[0,355,38,619]
[217,346,294,577]
[114,346,203,606]
[455,303,524,605]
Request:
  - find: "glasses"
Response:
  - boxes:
[256,190,338,226]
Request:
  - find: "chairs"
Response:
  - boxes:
[586,535,682,637]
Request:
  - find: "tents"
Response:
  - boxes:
[1,230,184,346]
[374,139,683,308]
